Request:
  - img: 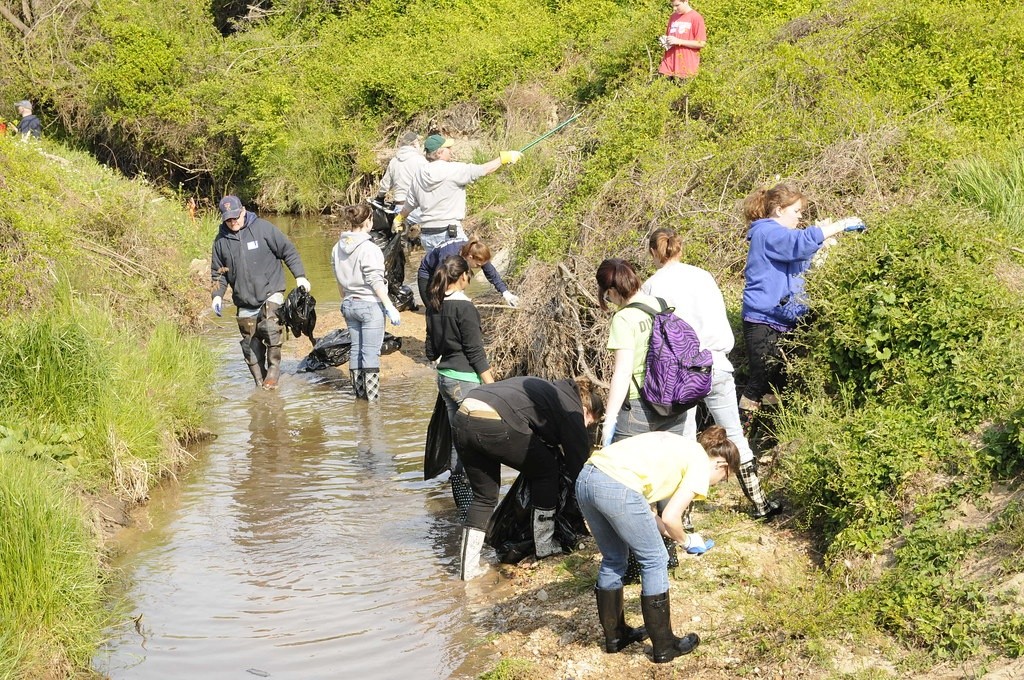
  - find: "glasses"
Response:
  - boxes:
[226,212,241,221]
[603,289,612,302]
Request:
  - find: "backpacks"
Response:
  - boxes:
[612,298,713,417]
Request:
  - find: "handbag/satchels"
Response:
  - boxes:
[424,392,452,480]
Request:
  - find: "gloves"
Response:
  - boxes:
[391,212,404,234]
[503,290,519,306]
[384,303,401,325]
[340,297,345,317]
[296,277,311,294]
[499,150,523,165]
[844,217,865,231]
[374,195,386,205]
[212,295,222,317]
[679,533,713,556]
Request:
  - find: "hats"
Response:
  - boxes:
[424,135,454,153]
[14,100,32,109]
[219,196,242,221]
[404,132,423,143]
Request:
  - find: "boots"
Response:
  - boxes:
[459,527,489,581]
[640,588,700,663]
[619,514,678,586]
[451,472,474,522]
[236,300,284,390]
[595,581,649,653]
[736,457,782,523]
[739,394,779,455]
[681,506,694,534]
[349,368,379,402]
[530,504,562,560]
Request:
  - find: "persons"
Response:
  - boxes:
[738,179,865,422]
[210,196,310,389]
[595,259,689,446]
[450,376,607,582]
[8,100,41,141]
[658,0,706,85]
[331,132,524,479]
[575,426,740,667]
[639,227,782,523]
